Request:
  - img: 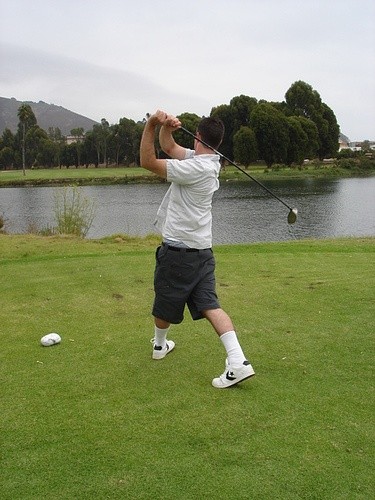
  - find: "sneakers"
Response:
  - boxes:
[212,359,256,387]
[151,337,175,359]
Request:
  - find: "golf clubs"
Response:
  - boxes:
[179,124,298,225]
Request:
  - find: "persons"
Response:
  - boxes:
[140,108,255,389]
